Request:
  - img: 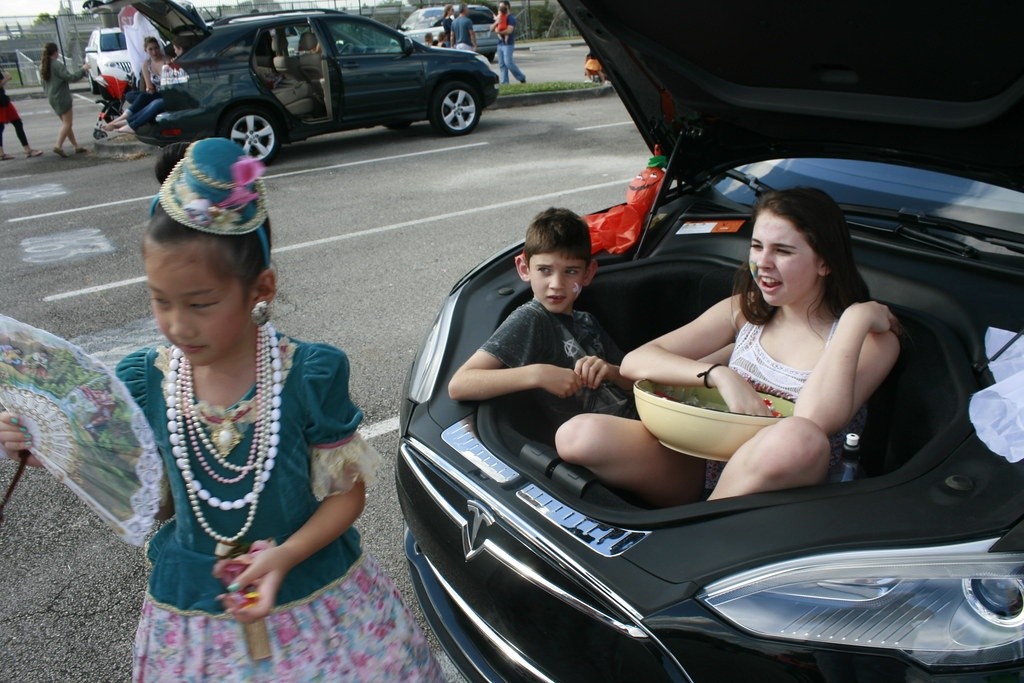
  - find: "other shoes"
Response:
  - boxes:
[583,79,593,83]
[74,148,88,153]
[520,76,526,83]
[53,146,68,158]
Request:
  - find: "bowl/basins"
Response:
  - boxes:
[633,377,796,462]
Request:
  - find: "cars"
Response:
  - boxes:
[84,25,137,100]
[394,4,501,64]
[392,1,1024,683]
[81,0,502,166]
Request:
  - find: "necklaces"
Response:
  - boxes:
[167,326,283,542]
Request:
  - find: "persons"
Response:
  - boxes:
[1,138,447,683]
[447,206,641,420]
[102,37,198,134]
[424,31,447,47]
[494,6,509,44]
[442,4,455,46]
[39,43,91,157]
[490,0,527,84]
[0,73,43,159]
[450,3,477,51]
[556,188,909,502]
[584,53,605,83]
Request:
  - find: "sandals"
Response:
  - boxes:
[24,149,43,159]
[0,154,15,161]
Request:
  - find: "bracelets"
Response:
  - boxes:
[696,364,722,389]
